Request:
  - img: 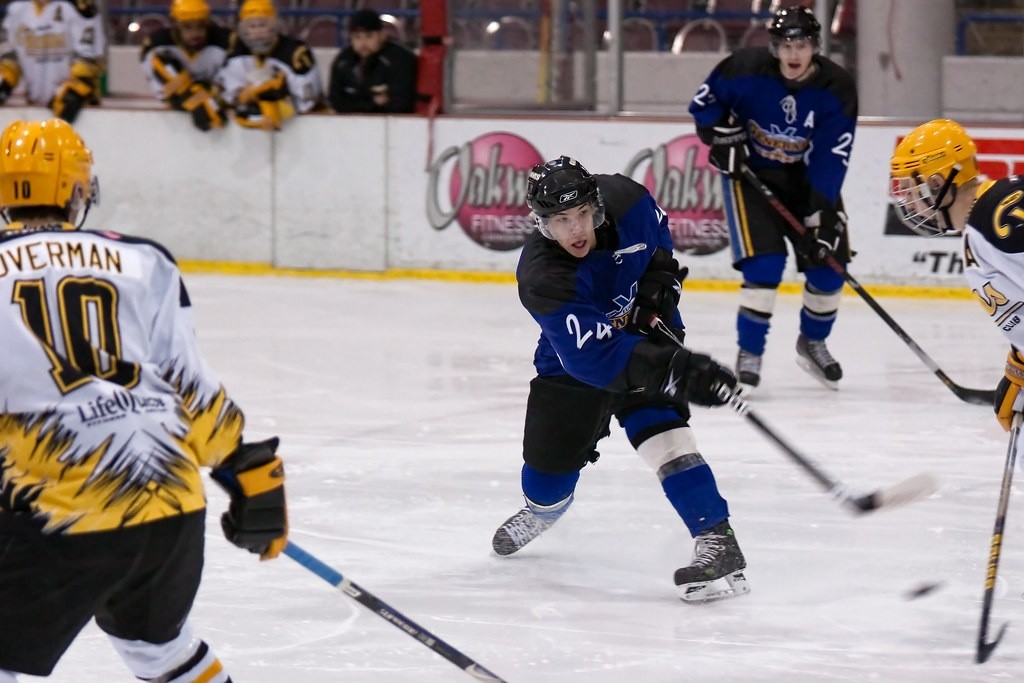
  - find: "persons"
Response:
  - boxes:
[688,3,858,388]
[140,0,323,131]
[492,155,747,585]
[327,10,420,114]
[0,118,290,683]
[0,0,107,123]
[891,119,1024,434]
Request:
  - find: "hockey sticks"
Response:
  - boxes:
[975,392,1024,664]
[219,508,507,683]
[736,161,996,408]
[649,311,945,517]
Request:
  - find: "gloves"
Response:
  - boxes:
[209,437,288,560]
[638,250,688,313]
[799,209,849,268]
[640,346,743,406]
[994,349,1024,432]
[151,56,294,132]
[0,58,19,106]
[709,108,751,178]
[46,61,98,123]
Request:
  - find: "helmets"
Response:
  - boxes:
[0,117,93,208]
[239,0,277,19]
[767,6,824,58]
[171,0,209,20]
[890,119,980,239]
[525,154,605,240]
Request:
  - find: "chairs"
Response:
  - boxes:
[107,0,857,55]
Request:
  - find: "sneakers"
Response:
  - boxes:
[736,348,762,395]
[675,521,750,601]
[795,333,842,391]
[489,494,579,555]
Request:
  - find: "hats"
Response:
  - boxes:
[348,9,383,31]
